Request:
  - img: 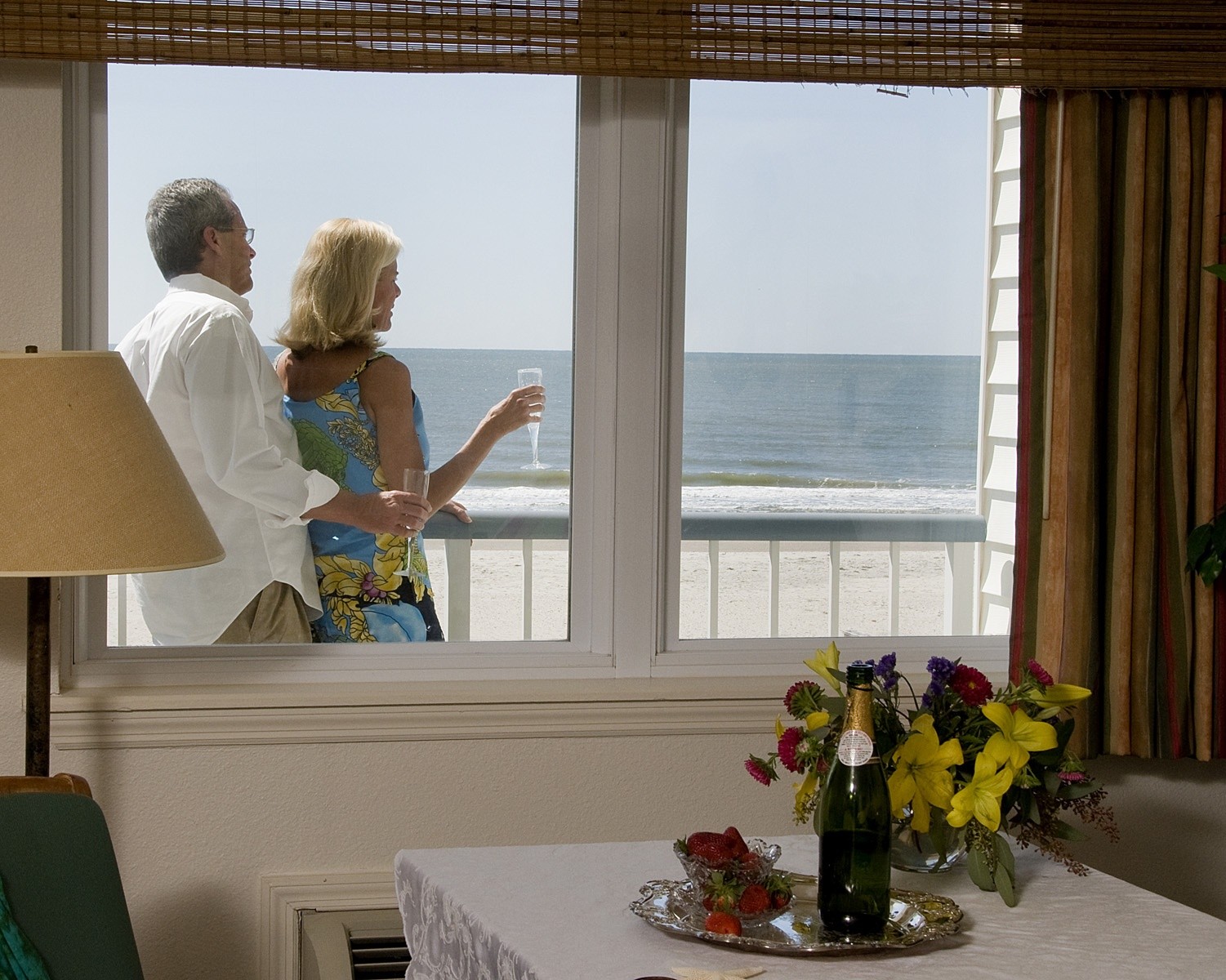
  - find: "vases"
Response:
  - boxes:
[892,807,971,872]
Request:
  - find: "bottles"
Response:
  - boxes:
[814,663,893,934]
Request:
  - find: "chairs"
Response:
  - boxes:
[0,774,146,980]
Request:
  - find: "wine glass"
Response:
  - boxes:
[517,367,554,470]
[388,467,428,578]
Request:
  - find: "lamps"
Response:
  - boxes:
[0,343,224,781]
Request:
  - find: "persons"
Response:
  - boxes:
[271,218,545,643]
[113,178,432,646]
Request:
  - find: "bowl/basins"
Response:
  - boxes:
[674,836,797,927]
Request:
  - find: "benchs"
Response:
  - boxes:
[1001,755,1226,923]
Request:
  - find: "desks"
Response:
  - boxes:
[391,818,1226,980]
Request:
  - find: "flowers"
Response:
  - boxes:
[743,642,1118,907]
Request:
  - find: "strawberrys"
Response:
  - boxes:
[676,825,794,938]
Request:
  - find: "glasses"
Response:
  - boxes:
[198,222,256,245]
